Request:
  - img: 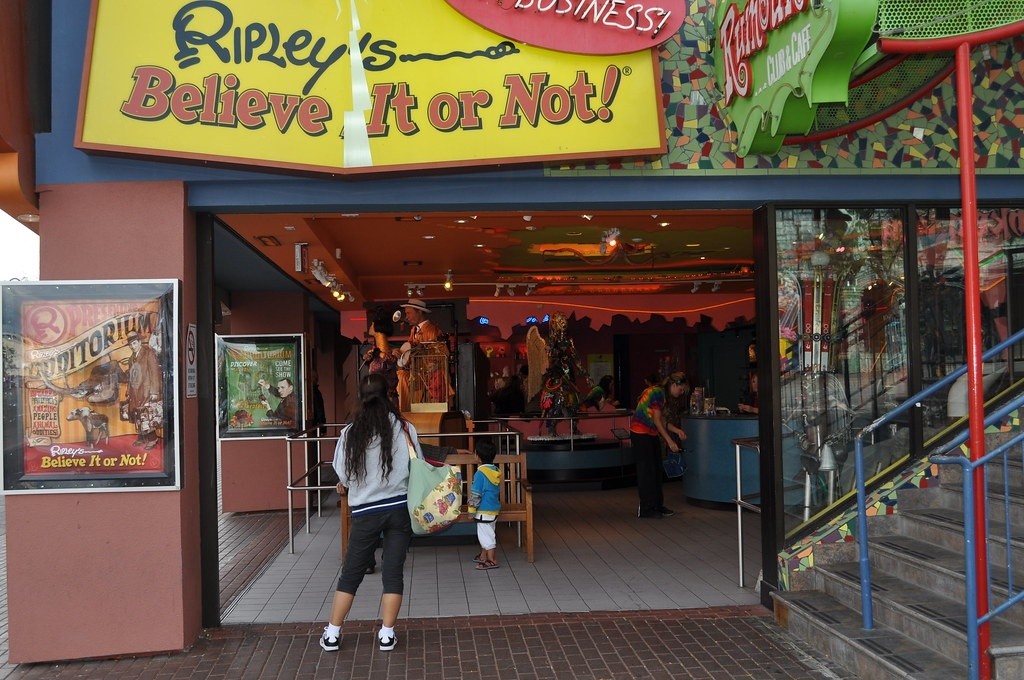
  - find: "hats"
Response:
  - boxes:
[400,299,431,313]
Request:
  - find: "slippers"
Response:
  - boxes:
[473,553,500,570]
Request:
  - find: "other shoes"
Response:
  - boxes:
[637,506,674,519]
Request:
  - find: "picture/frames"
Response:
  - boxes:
[217,333,305,441]
[0,278,181,495]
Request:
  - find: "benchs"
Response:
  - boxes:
[341,453,535,563]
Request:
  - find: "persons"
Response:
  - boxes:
[257,378,297,428]
[467,440,502,571]
[541,311,595,437]
[629,369,690,517]
[583,375,621,410]
[739,371,759,415]
[320,375,424,653]
[126,331,161,450]
[392,298,455,412]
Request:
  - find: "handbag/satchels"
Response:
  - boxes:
[402,419,461,535]
[663,449,688,478]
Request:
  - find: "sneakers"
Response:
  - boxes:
[378,629,397,650]
[320,626,339,651]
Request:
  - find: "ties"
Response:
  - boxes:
[414,326,419,338]
